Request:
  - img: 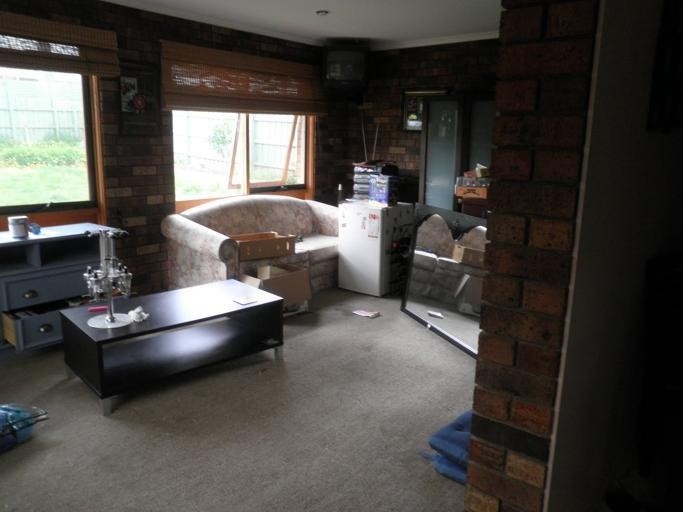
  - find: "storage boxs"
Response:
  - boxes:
[456,184,487,199]
[370,176,402,206]
[452,245,485,269]
[451,274,484,317]
[238,261,312,318]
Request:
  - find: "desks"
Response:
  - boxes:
[60,279,285,416]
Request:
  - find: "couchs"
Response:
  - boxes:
[159,194,340,293]
[408,204,486,317]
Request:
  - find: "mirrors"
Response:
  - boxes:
[399,202,487,358]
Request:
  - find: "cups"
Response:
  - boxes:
[84,270,133,303]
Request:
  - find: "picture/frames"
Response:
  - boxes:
[400,88,424,135]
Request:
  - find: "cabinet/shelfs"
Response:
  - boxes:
[457,188,494,219]
[0,223,130,353]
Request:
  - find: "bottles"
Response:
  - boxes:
[7,215,30,239]
[336,183,343,200]
[30,222,41,234]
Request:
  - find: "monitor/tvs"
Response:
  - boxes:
[321,47,370,88]
